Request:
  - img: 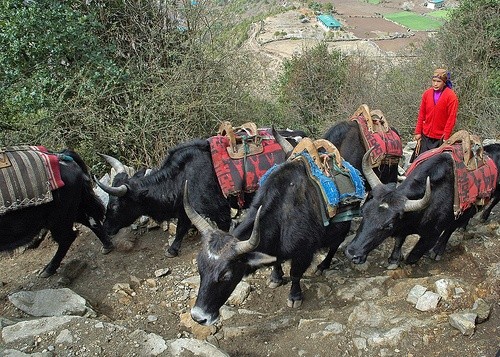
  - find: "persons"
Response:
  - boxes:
[409,68,459,163]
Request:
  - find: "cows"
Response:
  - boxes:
[94,128,308,258]
[344,143,500,270]
[183,119,403,326]
[0,144,114,278]
